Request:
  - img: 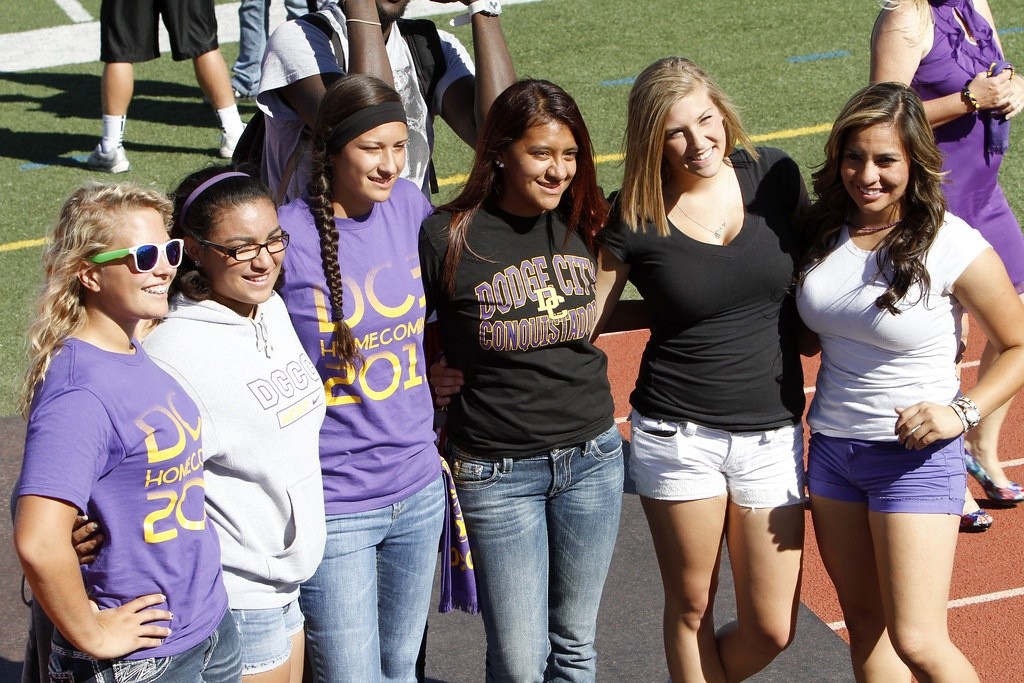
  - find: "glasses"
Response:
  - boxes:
[90,239,184,273]
[189,226,290,258]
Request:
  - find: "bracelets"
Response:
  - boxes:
[950,397,981,434]
[345,19,381,27]
[962,86,980,114]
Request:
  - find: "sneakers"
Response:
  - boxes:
[219,123,249,158]
[85,143,129,174]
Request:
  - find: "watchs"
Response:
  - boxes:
[468,0,502,18]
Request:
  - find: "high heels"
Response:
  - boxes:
[960,509,992,534]
[964,449,1023,503]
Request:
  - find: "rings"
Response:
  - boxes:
[435,386,440,395]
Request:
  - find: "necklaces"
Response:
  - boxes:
[675,175,730,238]
[853,218,901,230]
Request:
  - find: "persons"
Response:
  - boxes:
[256,0,517,212]
[795,83,1024,683]
[418,80,623,683]
[87,0,248,174]
[72,165,327,683]
[430,56,814,683]
[203,0,339,105]
[273,76,445,683]
[870,0,1024,533]
[10,180,242,683]
